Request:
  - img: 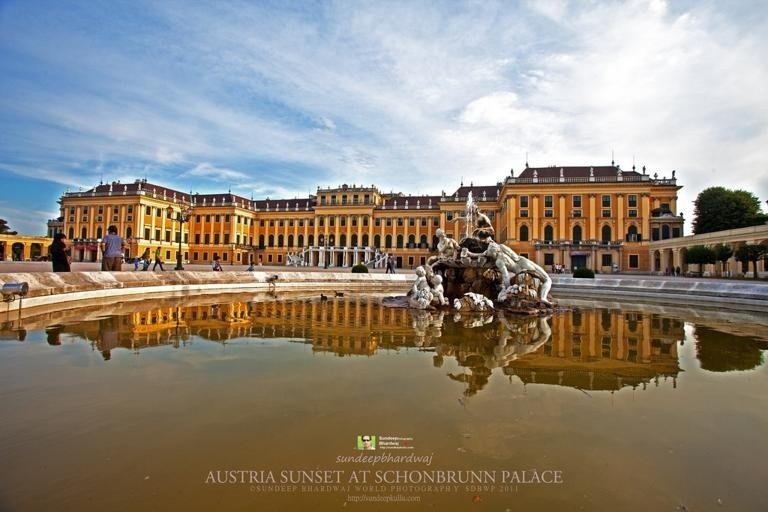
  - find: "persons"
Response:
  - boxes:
[51,234,70,271]
[46,324,64,345]
[671,266,675,277]
[213,256,222,271]
[676,266,680,277]
[247,262,255,271]
[553,263,565,274]
[386,257,392,274]
[134,247,165,271]
[96,319,117,361]
[410,311,553,396]
[390,258,395,273]
[101,225,126,271]
[409,205,552,311]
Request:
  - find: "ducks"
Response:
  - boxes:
[334,291,344,295]
[321,294,327,300]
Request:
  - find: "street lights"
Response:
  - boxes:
[166,194,195,271]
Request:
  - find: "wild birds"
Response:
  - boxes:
[265,275,278,288]
